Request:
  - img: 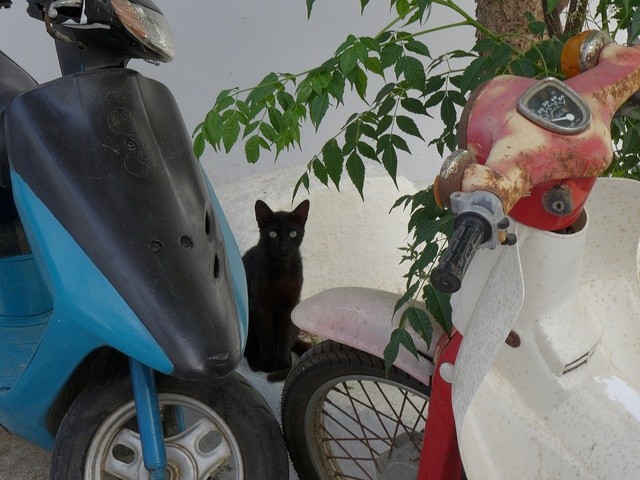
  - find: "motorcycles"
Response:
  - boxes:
[278,29,638,479]
[1,1,294,480]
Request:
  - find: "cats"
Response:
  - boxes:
[240,197,312,385]
